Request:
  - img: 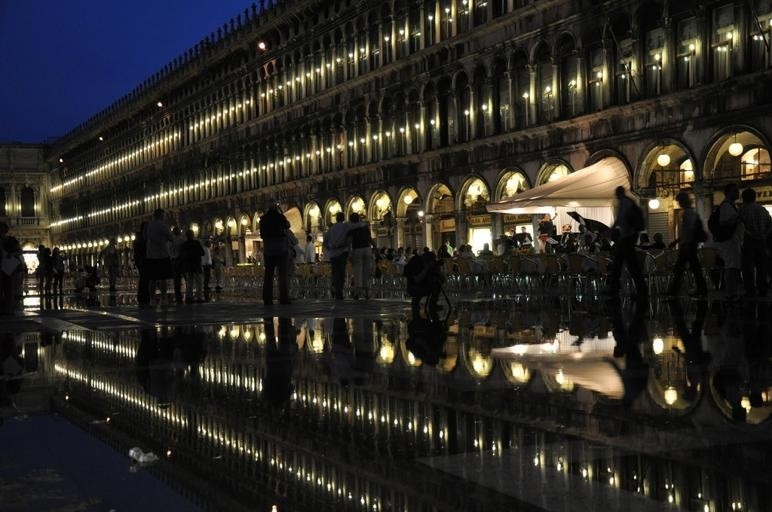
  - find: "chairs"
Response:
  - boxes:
[439,245,731,320]
[222,264,333,303]
[344,261,408,300]
[62,276,137,311]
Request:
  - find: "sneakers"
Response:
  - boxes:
[330,293,373,301]
[412,297,444,311]
[262,298,293,306]
[600,284,769,300]
[137,286,223,306]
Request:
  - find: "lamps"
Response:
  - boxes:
[657,140,671,168]
[213,187,412,231]
[728,132,744,157]
